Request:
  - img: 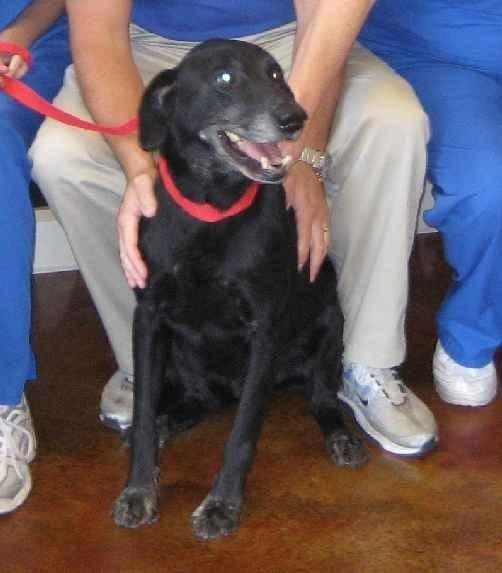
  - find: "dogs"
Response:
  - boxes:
[113,35,367,541]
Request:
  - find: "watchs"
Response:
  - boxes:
[296,147,334,172]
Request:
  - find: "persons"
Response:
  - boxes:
[23,0,443,463]
[359,0,502,410]
[2,0,89,513]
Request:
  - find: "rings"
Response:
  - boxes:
[321,224,332,232]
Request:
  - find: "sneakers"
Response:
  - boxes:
[430,333,500,409]
[95,366,134,433]
[0,392,38,519]
[331,349,443,460]
